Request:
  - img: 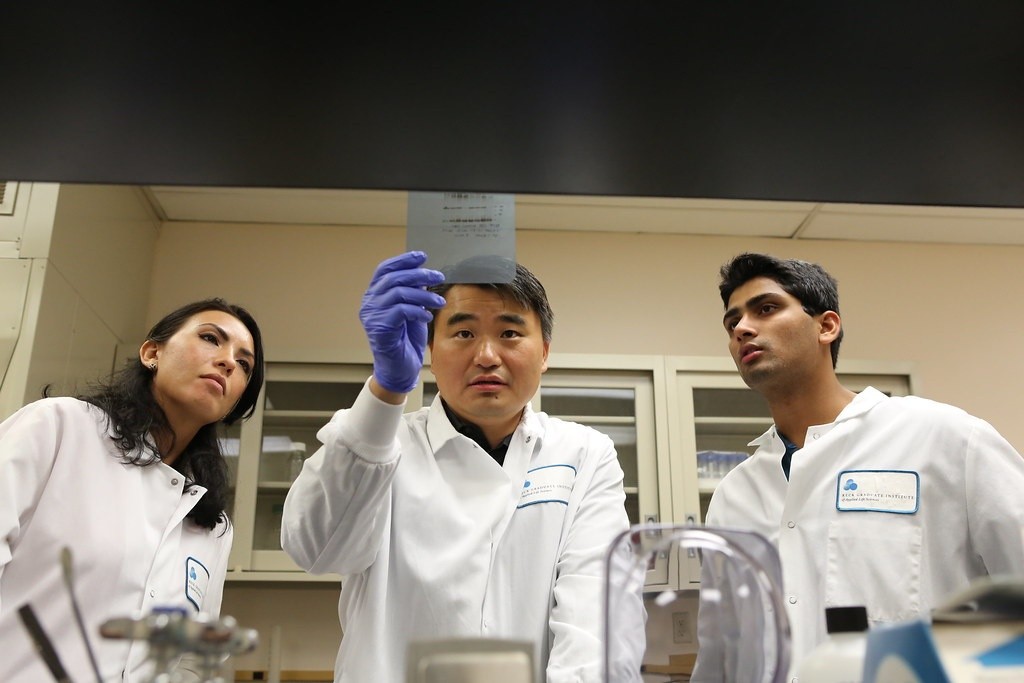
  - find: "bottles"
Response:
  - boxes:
[288,442,306,483]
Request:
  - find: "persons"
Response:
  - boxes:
[279,250,652,683]
[688,253,1023,683]
[0,299,266,683]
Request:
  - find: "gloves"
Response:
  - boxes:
[360,250,448,392]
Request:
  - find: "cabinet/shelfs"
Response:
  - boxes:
[100,340,936,581]
